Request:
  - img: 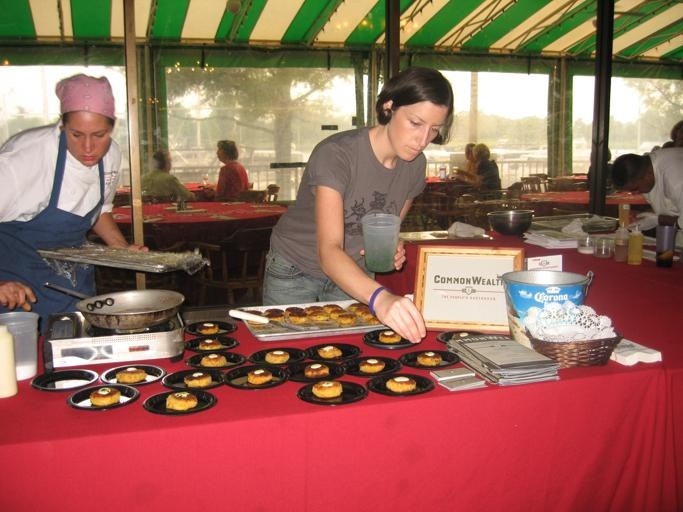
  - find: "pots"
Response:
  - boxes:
[44,281,185,328]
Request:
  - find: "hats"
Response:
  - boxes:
[56,75,116,118]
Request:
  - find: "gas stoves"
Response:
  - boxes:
[46,308,184,368]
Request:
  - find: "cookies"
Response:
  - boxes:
[362,312,373,319]
[325,305,341,312]
[387,376,414,393]
[319,347,341,358]
[184,373,212,388]
[307,306,322,313]
[265,351,289,363]
[350,304,365,312]
[304,363,329,378]
[360,358,384,374]
[418,351,440,367]
[380,330,402,344]
[330,310,347,319]
[355,307,369,314]
[313,382,341,400]
[309,312,328,320]
[200,340,221,350]
[248,370,272,385]
[267,313,284,320]
[117,369,145,384]
[286,308,303,313]
[90,388,120,406]
[369,318,379,324]
[339,314,355,323]
[165,393,197,410]
[198,323,220,334]
[266,309,283,314]
[203,355,226,366]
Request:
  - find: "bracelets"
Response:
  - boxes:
[368,286,389,317]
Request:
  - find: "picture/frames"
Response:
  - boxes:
[412,244,524,337]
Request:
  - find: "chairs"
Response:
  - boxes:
[522,176,542,192]
[190,226,273,305]
[235,189,266,202]
[260,183,281,203]
[530,173,550,192]
[87,233,185,295]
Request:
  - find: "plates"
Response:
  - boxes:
[400,349,458,369]
[186,332,239,351]
[363,329,415,349]
[31,368,97,390]
[187,349,245,371]
[225,364,285,388]
[239,299,395,340]
[100,364,162,386]
[368,371,436,395]
[248,346,305,367]
[185,321,236,336]
[142,390,217,415]
[161,367,225,391]
[297,379,366,405]
[284,359,344,382]
[437,328,480,344]
[39,243,203,273]
[303,343,362,365]
[344,356,399,376]
[68,383,139,410]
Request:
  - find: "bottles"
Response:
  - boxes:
[615,222,628,262]
[0,325,18,399]
[620,206,630,228]
[629,226,643,264]
[655,215,675,268]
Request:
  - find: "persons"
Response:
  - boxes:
[451,141,478,190]
[260,64,455,348]
[143,150,199,202]
[197,139,251,202]
[665,119,683,149]
[2,75,149,337]
[467,143,502,202]
[611,147,683,239]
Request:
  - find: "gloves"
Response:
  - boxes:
[628,213,658,232]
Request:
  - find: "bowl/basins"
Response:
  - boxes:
[488,209,534,236]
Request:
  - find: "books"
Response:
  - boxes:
[522,230,579,250]
[445,334,562,387]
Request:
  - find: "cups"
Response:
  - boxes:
[656,214,677,267]
[577,235,596,254]
[593,238,611,258]
[2,312,38,382]
[362,213,400,273]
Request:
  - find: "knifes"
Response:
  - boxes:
[230,307,303,333]
